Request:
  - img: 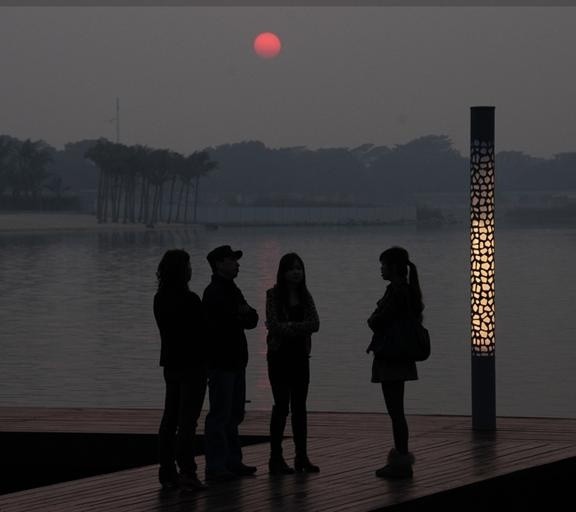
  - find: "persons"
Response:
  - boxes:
[264,253,320,478]
[366,247,423,479]
[153,248,210,493]
[201,244,258,485]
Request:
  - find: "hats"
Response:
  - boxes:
[207,244,242,265]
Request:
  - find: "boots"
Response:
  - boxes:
[291,414,319,473]
[376,448,414,479]
[270,405,294,474]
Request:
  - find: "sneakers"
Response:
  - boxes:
[160,464,256,490]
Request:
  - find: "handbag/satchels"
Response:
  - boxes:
[414,325,430,361]
